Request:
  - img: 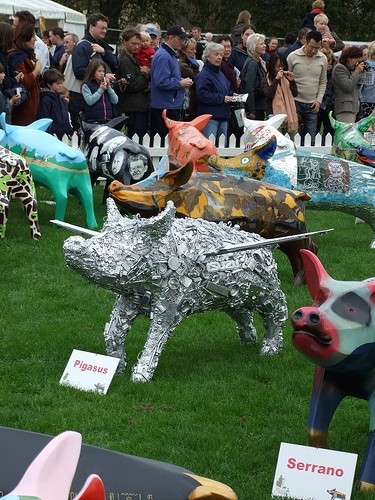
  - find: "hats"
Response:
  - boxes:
[167,25,192,39]
[140,24,158,36]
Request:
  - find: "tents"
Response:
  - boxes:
[1,0,87,49]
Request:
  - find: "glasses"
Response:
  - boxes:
[275,64,284,70]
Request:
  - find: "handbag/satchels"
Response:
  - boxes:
[62,39,96,93]
[5,83,29,107]
[234,98,247,127]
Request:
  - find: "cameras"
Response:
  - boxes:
[13,87,20,97]
[356,61,371,72]
[101,77,106,85]
[282,71,289,76]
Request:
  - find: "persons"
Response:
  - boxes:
[58,86,74,128]
[80,57,119,125]
[285,30,328,145]
[331,45,365,123]
[0,0,375,116]
[259,54,299,142]
[149,24,194,149]
[355,40,375,123]
[37,68,76,141]
[315,47,336,145]
[0,22,43,129]
[193,42,240,159]
[62,12,114,148]
[238,32,268,122]
[217,36,244,159]
[117,29,151,144]
